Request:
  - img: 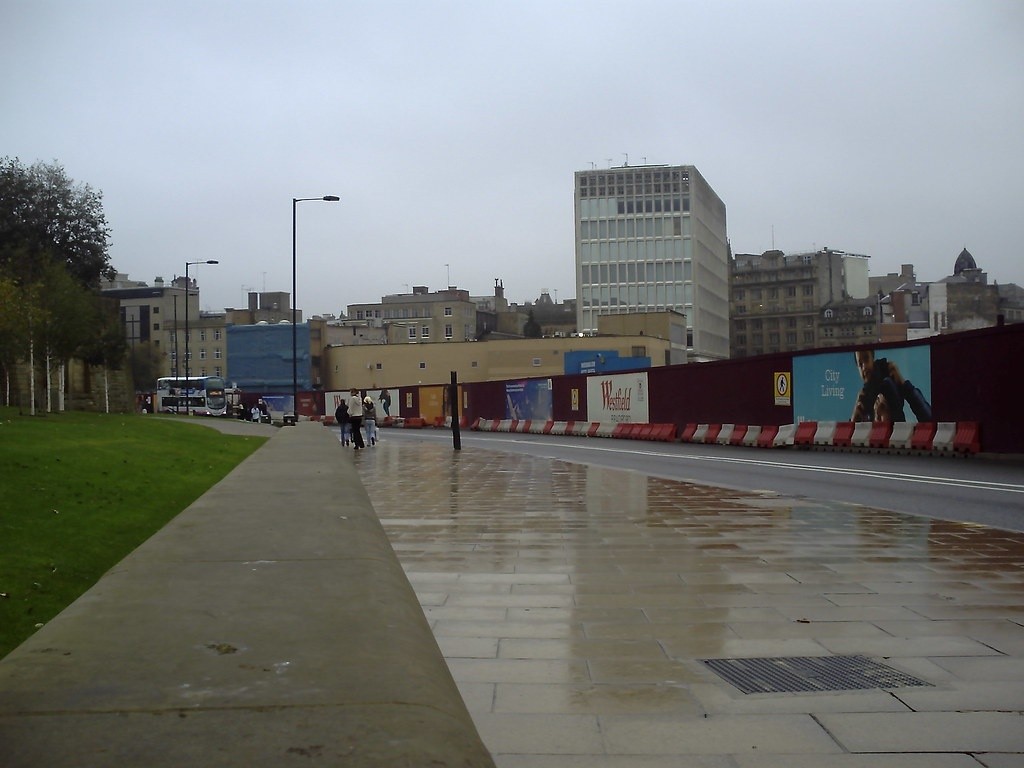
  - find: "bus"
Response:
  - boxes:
[156,376,227,418]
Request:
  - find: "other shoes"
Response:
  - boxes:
[354,446,359,450]
[371,437,375,445]
[346,439,349,446]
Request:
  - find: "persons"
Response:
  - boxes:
[347,388,365,450]
[362,396,376,446]
[227,400,260,423]
[377,390,391,416]
[335,399,351,446]
[851,350,931,422]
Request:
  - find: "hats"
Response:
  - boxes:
[364,396,371,404]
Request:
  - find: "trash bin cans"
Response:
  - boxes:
[283,414,295,426]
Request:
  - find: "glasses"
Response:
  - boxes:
[351,391,354,393]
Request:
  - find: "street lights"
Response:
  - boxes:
[442,263,451,286]
[292,195,341,417]
[184,260,220,416]
